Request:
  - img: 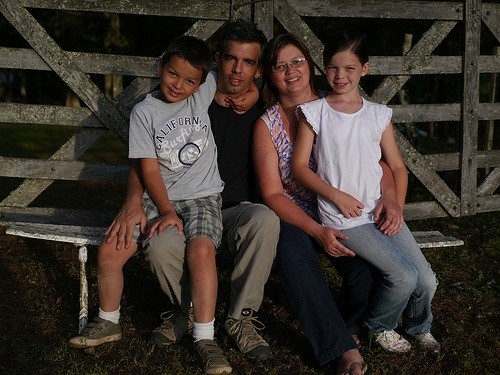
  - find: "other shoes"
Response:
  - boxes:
[374,330,412,353]
[414,332,440,354]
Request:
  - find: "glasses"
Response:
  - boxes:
[272,58,307,73]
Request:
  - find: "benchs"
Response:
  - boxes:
[6,224,465,336]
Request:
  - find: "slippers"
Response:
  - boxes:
[337,362,368,375]
[351,335,361,350]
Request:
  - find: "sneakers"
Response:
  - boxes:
[194,339,232,374]
[151,309,188,346]
[68,317,122,348]
[224,308,272,362]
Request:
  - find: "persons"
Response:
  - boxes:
[68,34,259,375]
[251,33,403,375]
[104,19,269,362]
[291,29,441,352]
